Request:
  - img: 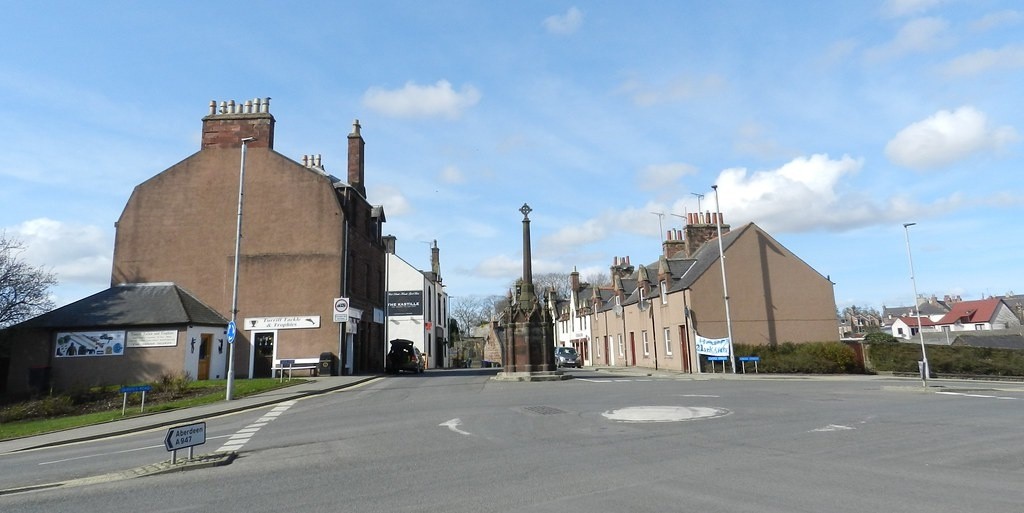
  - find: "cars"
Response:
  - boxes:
[555,347,581,368]
[386,339,426,374]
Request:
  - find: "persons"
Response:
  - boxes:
[390,351,401,375]
[467,357,472,367]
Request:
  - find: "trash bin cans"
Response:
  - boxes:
[318,351,333,377]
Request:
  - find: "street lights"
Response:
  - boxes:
[904,223,931,379]
[226,137,257,401]
[711,185,736,373]
